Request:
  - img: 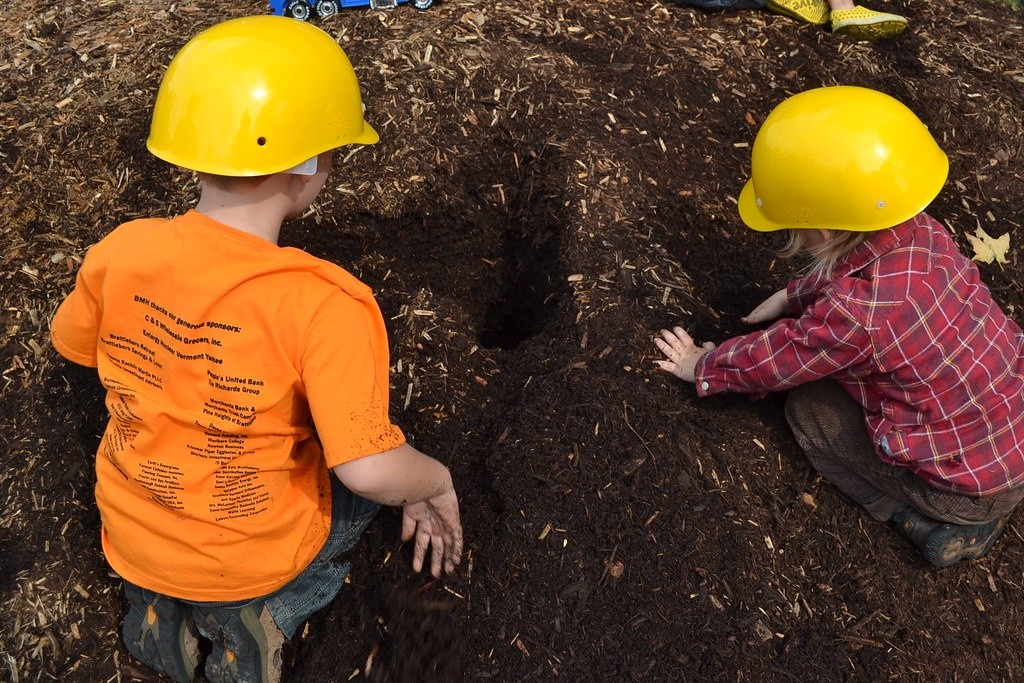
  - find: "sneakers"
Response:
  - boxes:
[123,578,203,683]
[193,601,286,683]
[893,505,1007,569]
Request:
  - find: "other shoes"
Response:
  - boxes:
[829,5,908,39]
[767,0,829,25]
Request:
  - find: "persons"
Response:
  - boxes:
[653,86,1024,566]
[693,0,908,40]
[50,15,463,683]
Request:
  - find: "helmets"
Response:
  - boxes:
[146,16,379,177]
[737,86,949,233]
